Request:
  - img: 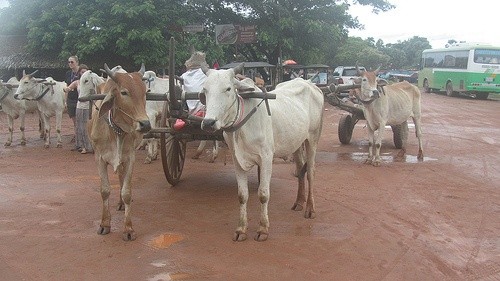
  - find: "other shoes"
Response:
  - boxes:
[81,149,87,154]
[71,146,76,151]
[70,137,76,143]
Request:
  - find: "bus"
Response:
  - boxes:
[419,45,500,99]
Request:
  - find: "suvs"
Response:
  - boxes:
[330,65,368,85]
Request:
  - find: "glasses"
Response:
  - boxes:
[69,61,74,63]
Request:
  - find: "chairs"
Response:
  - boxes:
[476,57,499,64]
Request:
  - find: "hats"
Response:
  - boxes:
[185,51,206,68]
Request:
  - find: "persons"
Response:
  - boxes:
[63,56,80,126]
[174,51,209,129]
[65,65,94,154]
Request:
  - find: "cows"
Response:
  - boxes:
[354,64,424,166]
[199,63,324,243]
[87,62,150,240]
[0,69,218,164]
